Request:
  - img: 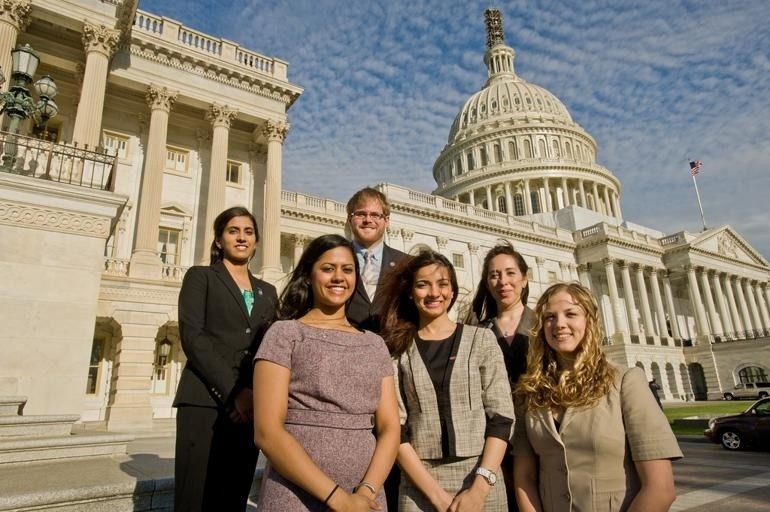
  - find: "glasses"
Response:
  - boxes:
[350,210,385,219]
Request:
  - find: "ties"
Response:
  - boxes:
[360,250,377,303]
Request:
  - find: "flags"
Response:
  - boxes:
[689,159,704,177]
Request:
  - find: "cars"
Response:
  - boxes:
[722,382,770,401]
[703,397,770,451]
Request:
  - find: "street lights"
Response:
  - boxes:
[0,43,61,174]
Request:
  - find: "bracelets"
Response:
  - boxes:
[355,481,379,503]
[324,483,341,506]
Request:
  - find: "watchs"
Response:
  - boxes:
[475,466,499,488]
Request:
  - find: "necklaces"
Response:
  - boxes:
[494,308,525,338]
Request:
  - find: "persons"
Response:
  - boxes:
[511,280,685,512]
[647,378,663,410]
[252,233,402,512]
[342,185,421,512]
[462,237,540,512]
[370,243,517,512]
[171,205,281,511]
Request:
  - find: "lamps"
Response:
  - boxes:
[159,327,171,357]
